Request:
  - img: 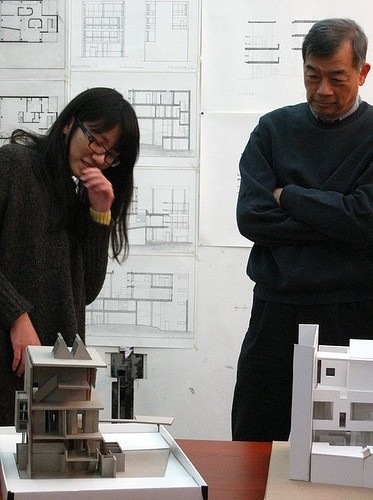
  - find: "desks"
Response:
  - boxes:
[173,436,289,500]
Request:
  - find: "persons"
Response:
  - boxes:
[229,17,372,442]
[0,87,140,431]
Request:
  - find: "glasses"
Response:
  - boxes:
[76,118,122,168]
